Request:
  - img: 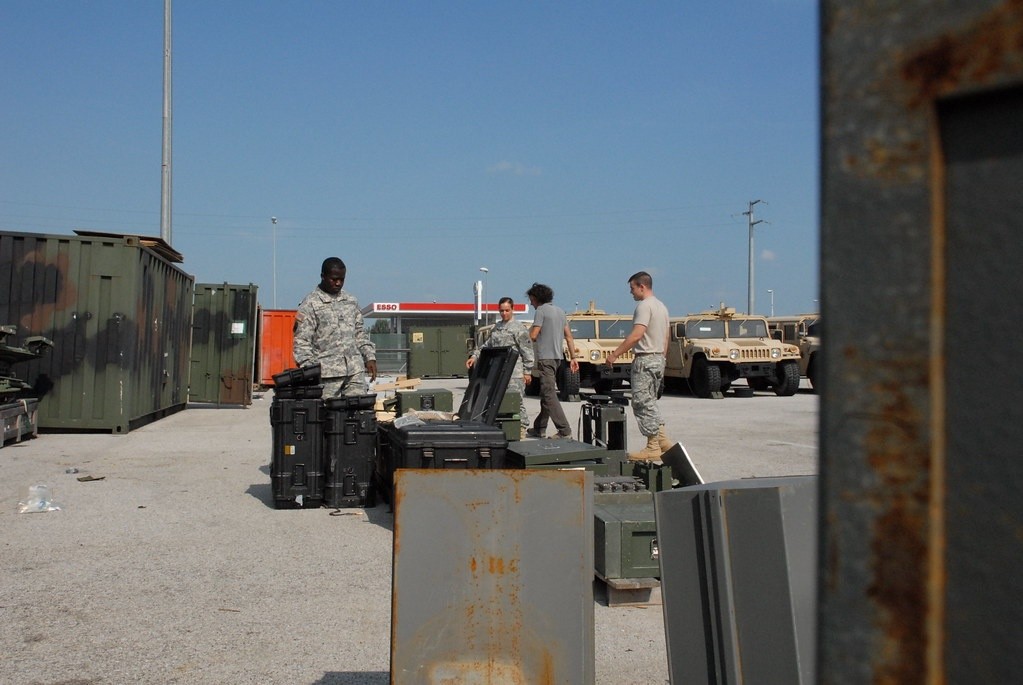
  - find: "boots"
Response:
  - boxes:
[627,435,662,461]
[658,425,672,452]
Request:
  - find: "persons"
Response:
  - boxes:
[606,270,673,461]
[526,282,579,441]
[466,296,535,439]
[292,256,377,406]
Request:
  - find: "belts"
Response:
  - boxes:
[635,352,664,356]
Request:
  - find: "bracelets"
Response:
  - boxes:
[571,355,576,359]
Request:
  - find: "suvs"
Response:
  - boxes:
[664,303,801,399]
[525,301,664,401]
[765,315,821,392]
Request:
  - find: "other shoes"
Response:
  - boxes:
[520,427,527,439]
[548,432,572,440]
[527,428,546,438]
